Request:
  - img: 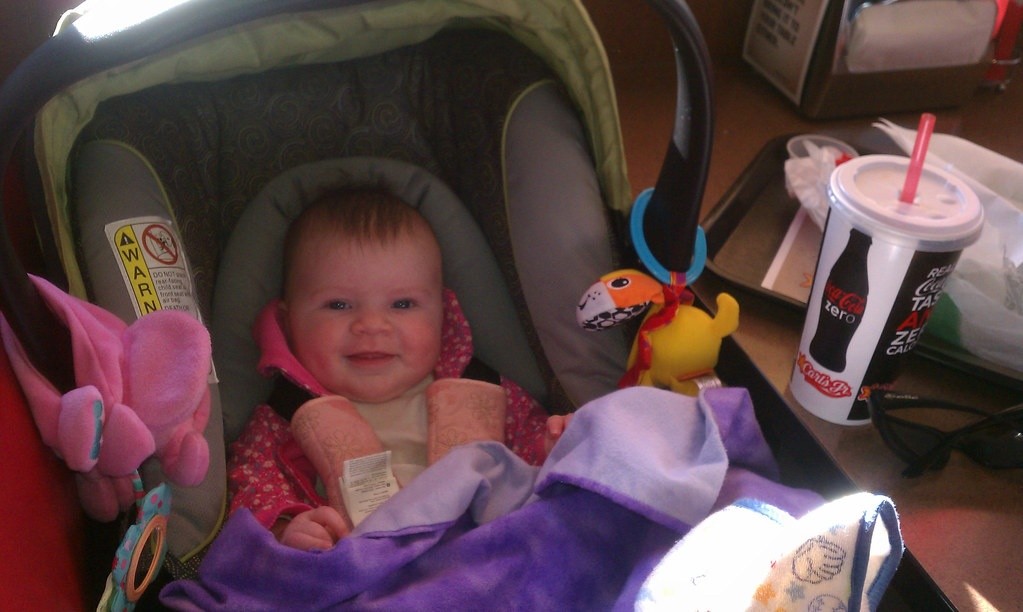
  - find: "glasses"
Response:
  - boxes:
[868,388,1023,478]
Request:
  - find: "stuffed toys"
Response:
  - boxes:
[577,270,740,396]
[0,270,213,520]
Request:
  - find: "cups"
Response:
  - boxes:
[788,152,985,425]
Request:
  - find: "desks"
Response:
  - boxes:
[587,0,1023,612]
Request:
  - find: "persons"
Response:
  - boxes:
[228,180,574,552]
